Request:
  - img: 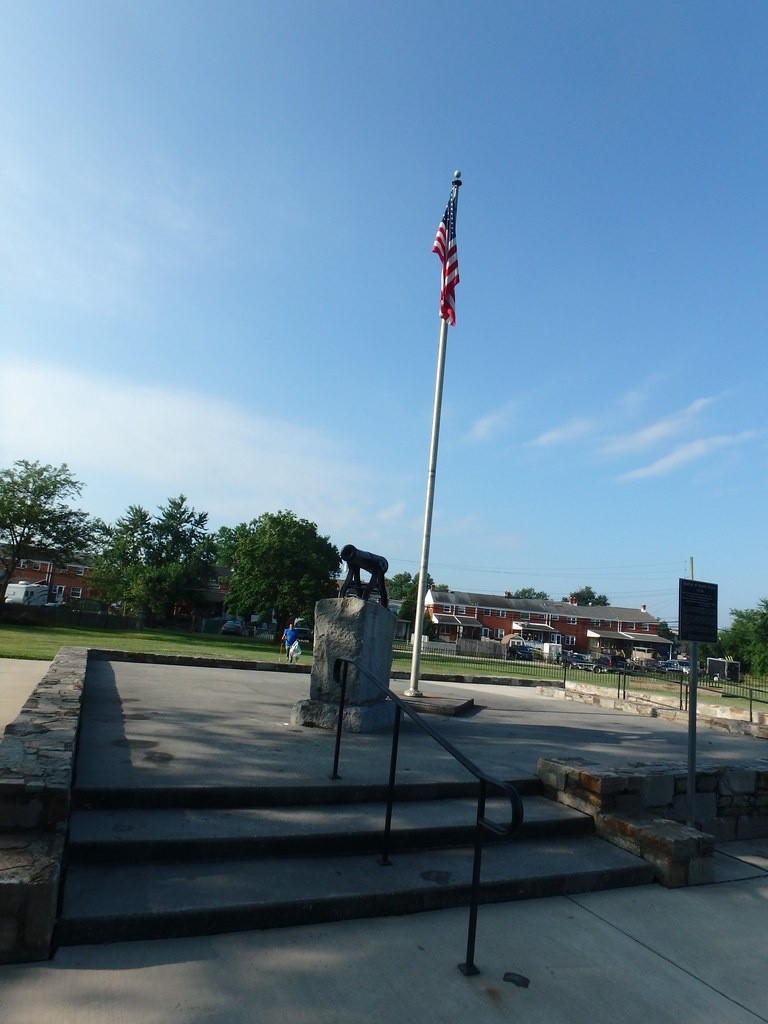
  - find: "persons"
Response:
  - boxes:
[281,623,299,663]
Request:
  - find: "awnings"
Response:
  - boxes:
[433,614,482,627]
[513,622,559,644]
[587,630,673,660]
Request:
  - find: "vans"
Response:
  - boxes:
[596,654,627,667]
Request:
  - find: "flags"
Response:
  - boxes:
[431,187,460,327]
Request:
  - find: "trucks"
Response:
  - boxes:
[570,660,634,676]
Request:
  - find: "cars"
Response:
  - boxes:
[222,621,243,636]
[561,654,594,670]
[507,645,534,661]
[294,627,313,643]
[667,659,690,675]
[658,660,681,673]
